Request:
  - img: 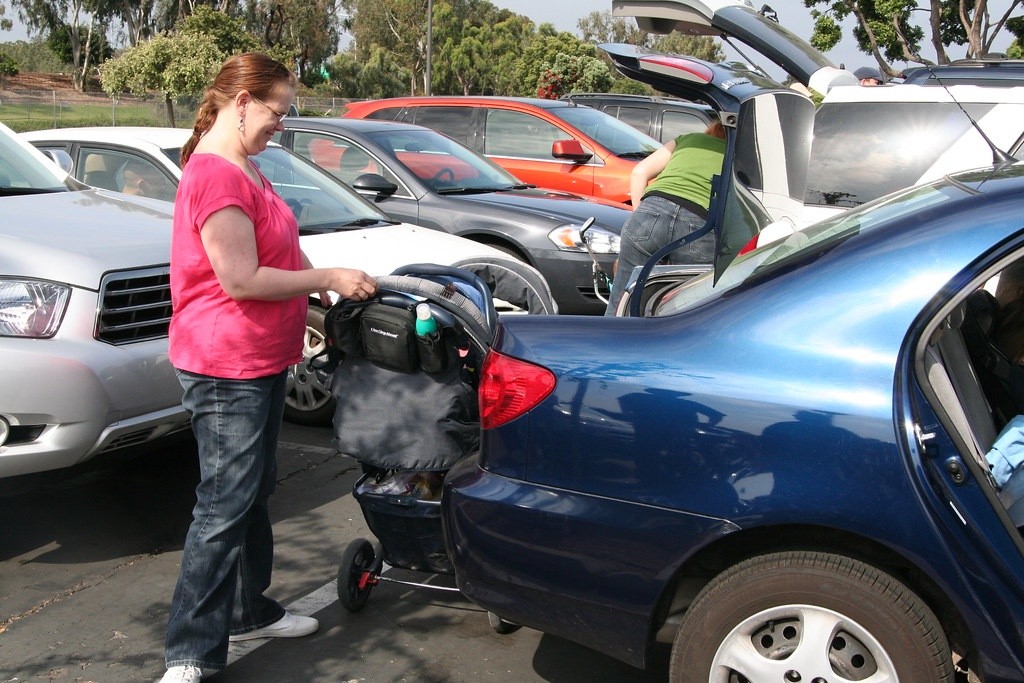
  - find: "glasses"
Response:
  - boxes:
[251,95,287,122]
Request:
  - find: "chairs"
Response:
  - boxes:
[341,146,370,186]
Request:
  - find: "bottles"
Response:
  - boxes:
[416,303,438,338]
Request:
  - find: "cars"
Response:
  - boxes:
[263,118,633,316]
[12,127,558,427]
[341,96,666,213]
[563,0,1024,245]
[0,122,194,479]
[440,160,1024,682]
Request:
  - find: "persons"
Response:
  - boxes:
[122,160,163,199]
[157,52,378,683]
[854,67,891,86]
[103,154,123,178]
[604,121,727,318]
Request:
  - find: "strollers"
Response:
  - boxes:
[308,262,560,634]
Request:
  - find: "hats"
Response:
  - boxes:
[853,66,883,81]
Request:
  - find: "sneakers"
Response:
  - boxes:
[229,611,319,642]
[158,666,202,683]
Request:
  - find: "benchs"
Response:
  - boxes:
[918,290,1024,527]
